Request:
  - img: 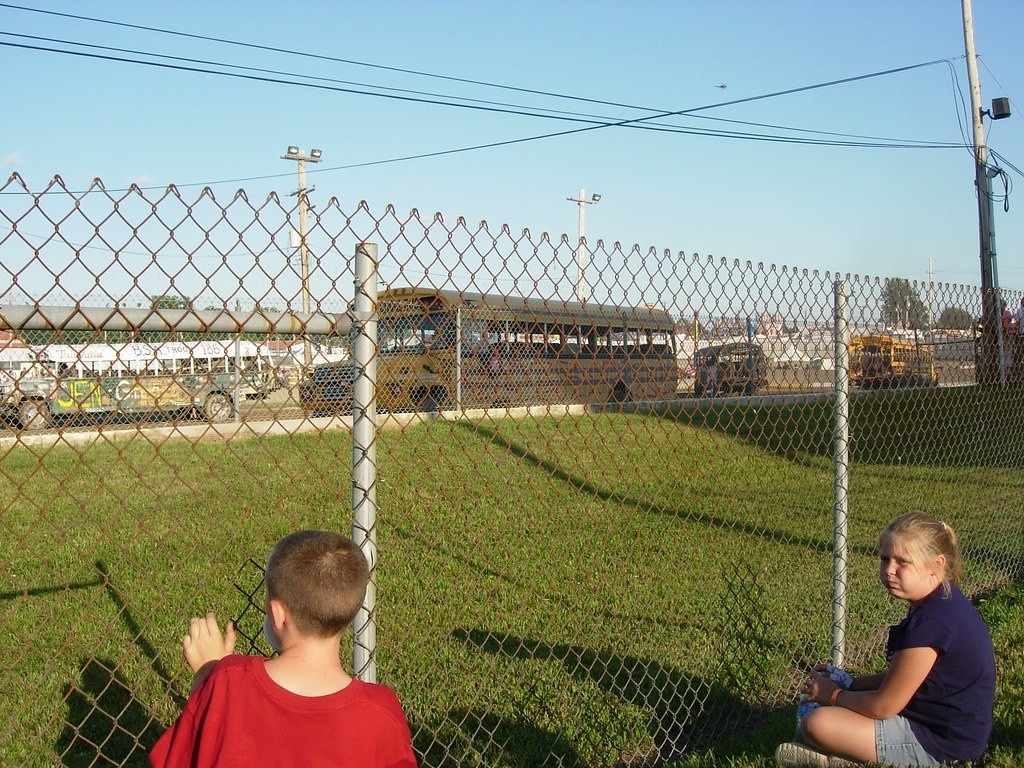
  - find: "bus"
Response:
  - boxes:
[298,287,680,416]
[687,342,769,397]
[0,340,278,432]
[845,335,943,391]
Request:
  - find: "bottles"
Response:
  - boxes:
[793,671,822,749]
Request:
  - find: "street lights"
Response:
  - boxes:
[277,145,325,373]
[970,97,1014,388]
[565,187,602,303]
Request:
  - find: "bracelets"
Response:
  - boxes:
[830,689,843,706]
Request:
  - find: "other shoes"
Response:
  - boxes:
[774,742,865,768]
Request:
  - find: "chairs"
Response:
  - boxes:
[489,341,672,356]
[64,368,221,378]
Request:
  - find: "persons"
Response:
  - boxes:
[774,512,996,768]
[148,531,418,768]
